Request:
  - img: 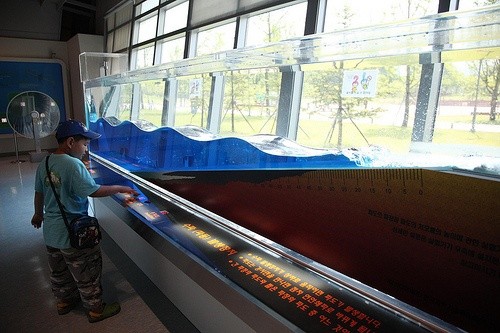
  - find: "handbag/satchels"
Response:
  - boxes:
[68,214,102,249]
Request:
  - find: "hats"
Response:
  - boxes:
[56,119,102,141]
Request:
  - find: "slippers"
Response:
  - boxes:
[56,298,81,316]
[88,301,121,322]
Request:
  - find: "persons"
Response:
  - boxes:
[30,119,137,324]
